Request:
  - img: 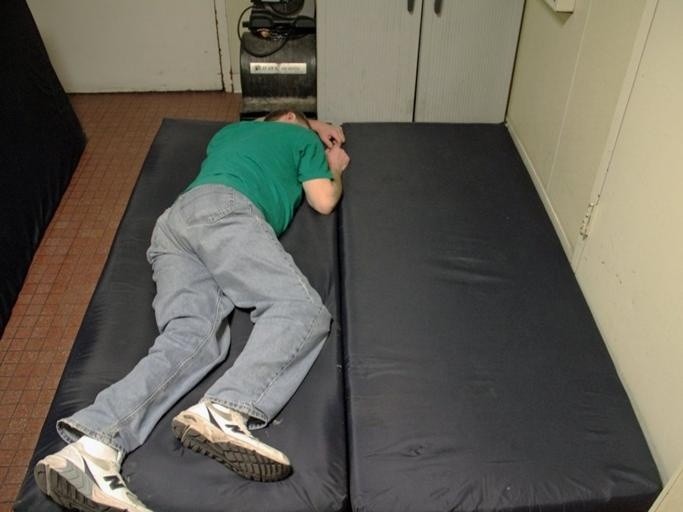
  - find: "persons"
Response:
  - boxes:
[32,109,351,511]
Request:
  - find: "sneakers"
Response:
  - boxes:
[172,398,293,482]
[35,442,155,511]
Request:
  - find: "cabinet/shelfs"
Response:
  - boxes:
[314,1,528,126]
